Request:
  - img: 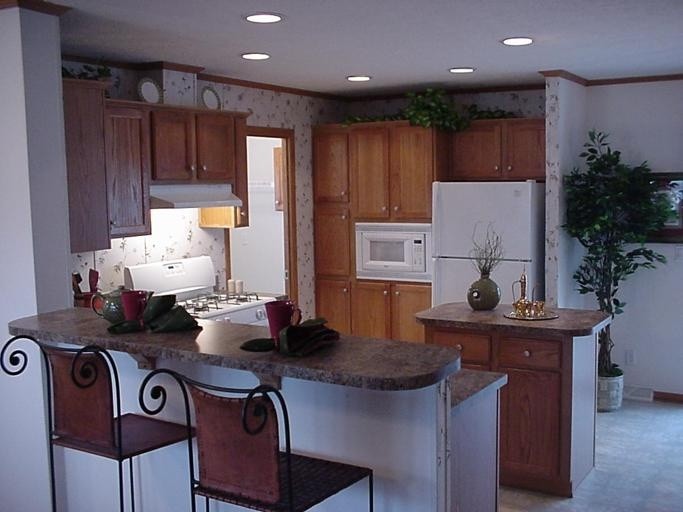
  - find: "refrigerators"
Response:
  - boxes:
[432,179,542,308]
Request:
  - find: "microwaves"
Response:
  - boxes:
[354,222,432,286]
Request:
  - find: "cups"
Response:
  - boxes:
[263,301,302,340]
[119,291,148,328]
[532,299,545,317]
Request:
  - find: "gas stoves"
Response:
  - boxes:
[171,286,277,321]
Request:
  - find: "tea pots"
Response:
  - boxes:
[88,287,155,335]
[512,273,538,319]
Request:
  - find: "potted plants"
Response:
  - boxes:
[555,126,679,410]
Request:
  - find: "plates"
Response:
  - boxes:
[137,77,163,105]
[503,308,560,322]
[200,85,221,112]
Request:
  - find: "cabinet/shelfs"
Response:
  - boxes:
[496,333,597,497]
[450,119,545,182]
[351,281,431,344]
[105,107,152,239]
[61,84,111,253]
[198,114,248,228]
[147,110,235,185]
[348,122,449,223]
[312,126,351,336]
[427,325,490,371]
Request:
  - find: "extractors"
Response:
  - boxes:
[147,183,243,212]
